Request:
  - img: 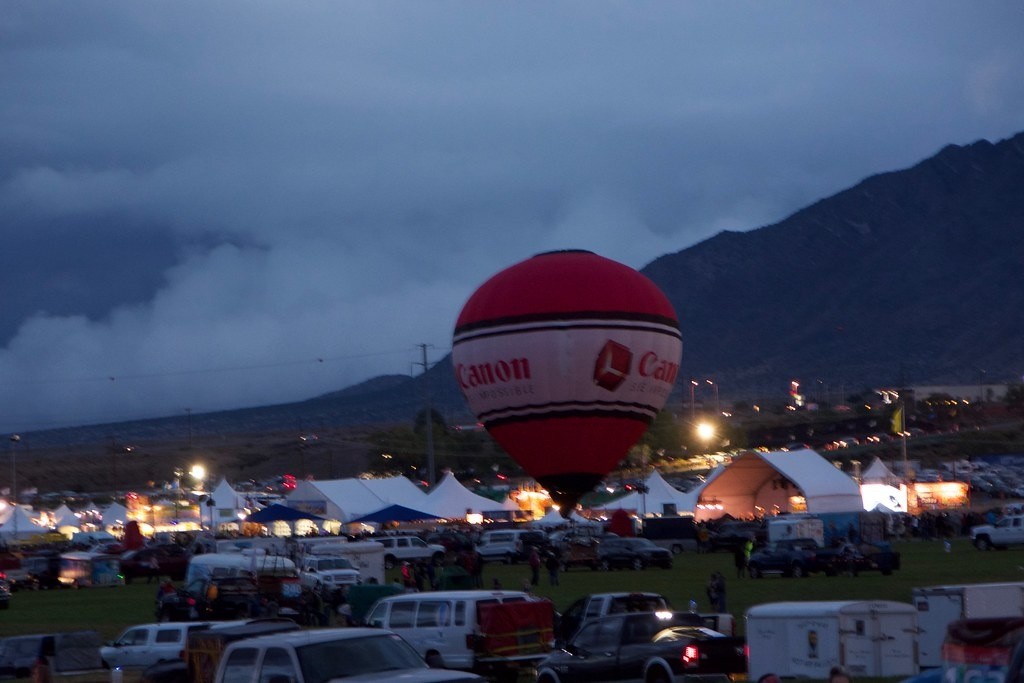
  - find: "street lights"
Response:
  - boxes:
[104,434,119,500]
[183,408,194,449]
[9,434,22,540]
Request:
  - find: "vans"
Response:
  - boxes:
[356,589,531,673]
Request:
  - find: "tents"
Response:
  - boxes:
[0,449,906,551]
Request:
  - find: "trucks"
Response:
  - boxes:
[637,515,720,555]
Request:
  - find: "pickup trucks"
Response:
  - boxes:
[534,610,749,683]
[143,626,489,683]
[556,592,736,653]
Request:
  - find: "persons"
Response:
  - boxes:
[888,507,1024,541]
[147,509,791,625]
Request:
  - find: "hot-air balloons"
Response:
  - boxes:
[450,249,684,529]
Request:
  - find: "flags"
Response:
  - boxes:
[888,408,903,433]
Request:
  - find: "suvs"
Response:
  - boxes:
[969,516,1024,552]
[595,537,673,572]
[96,619,216,669]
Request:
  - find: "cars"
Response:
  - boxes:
[714,520,768,552]
[748,536,847,581]
[0,529,627,623]
